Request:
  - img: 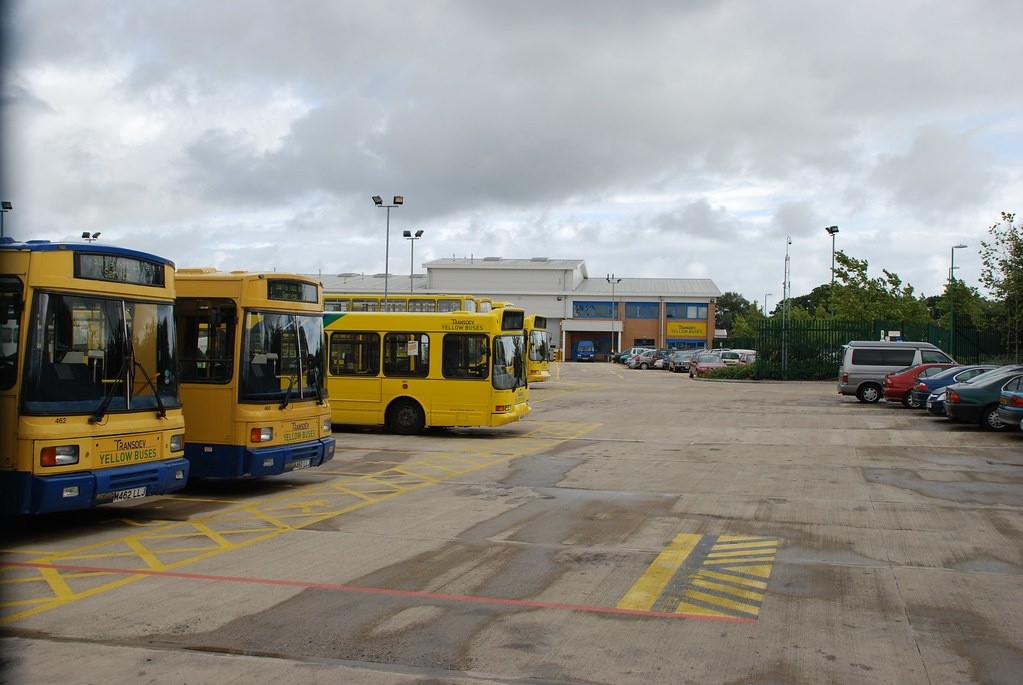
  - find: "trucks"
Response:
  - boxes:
[576,341,597,362]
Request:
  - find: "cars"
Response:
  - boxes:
[945,371,1023,432]
[926,365,1023,417]
[997,376,1023,434]
[912,365,1004,406]
[737,353,760,367]
[694,349,756,366]
[689,353,728,378]
[881,363,964,409]
[615,346,695,373]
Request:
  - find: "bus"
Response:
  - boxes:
[282,292,514,376]
[0,236,191,518]
[196,307,533,436]
[524,315,550,382]
[73,268,337,481]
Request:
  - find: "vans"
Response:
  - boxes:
[837,341,970,405]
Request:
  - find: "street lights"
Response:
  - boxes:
[372,195,404,312]
[82,232,101,243]
[0,201,13,237]
[951,244,968,277]
[825,225,839,317]
[781,236,793,378]
[606,278,621,363]
[403,230,424,294]
[949,267,960,284]
[765,294,773,316]
